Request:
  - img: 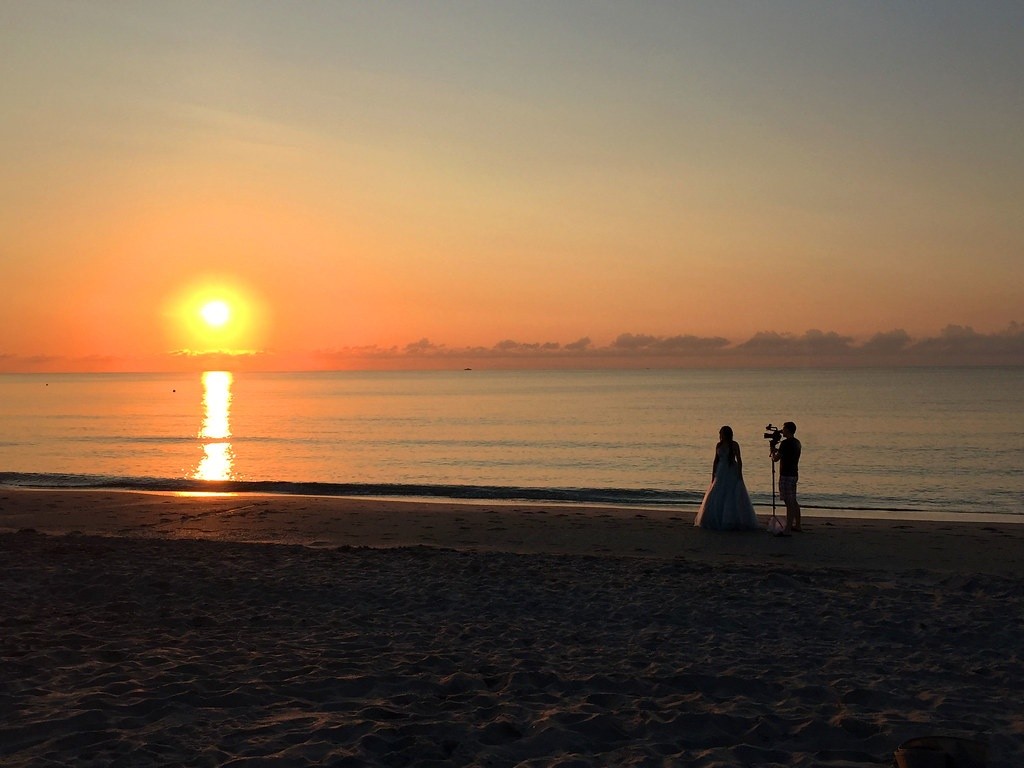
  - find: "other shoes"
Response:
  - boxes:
[774,531,793,537]
[792,526,802,532]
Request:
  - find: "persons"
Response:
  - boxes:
[768,422,803,538]
[710,424,744,530]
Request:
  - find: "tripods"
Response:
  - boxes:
[767,449,784,529]
